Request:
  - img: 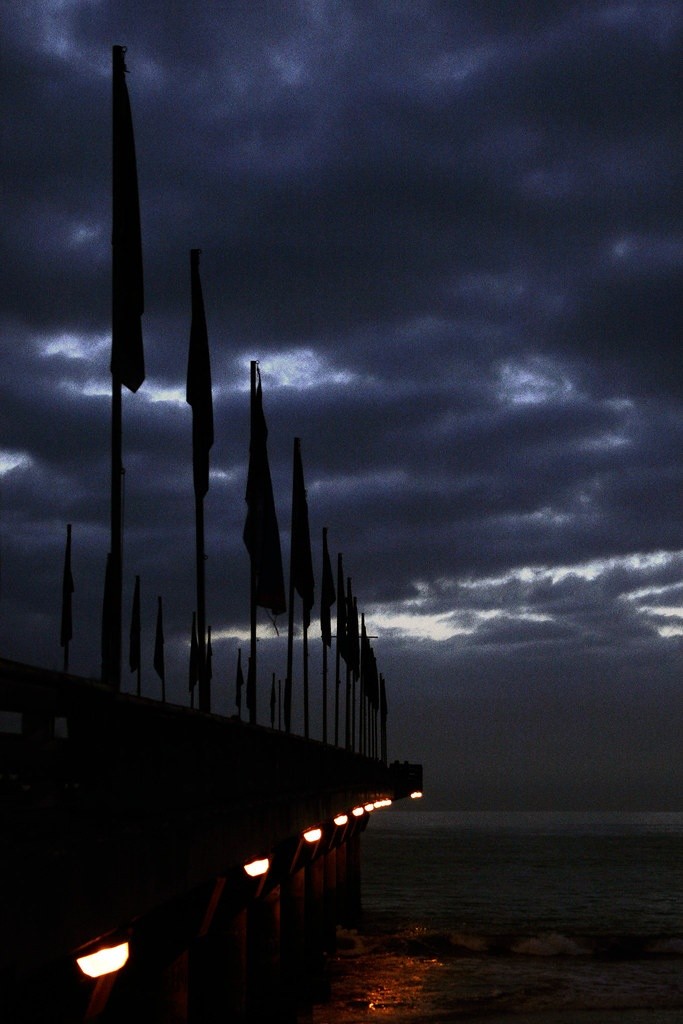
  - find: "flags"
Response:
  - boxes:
[54,255,387,720]
[113,72,146,393]
